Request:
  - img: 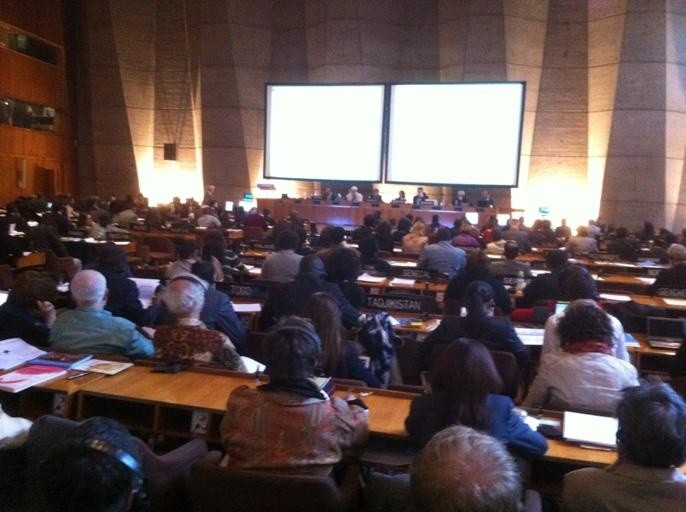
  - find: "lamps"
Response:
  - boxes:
[163,142,176,161]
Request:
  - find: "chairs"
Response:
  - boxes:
[0,219,686,511]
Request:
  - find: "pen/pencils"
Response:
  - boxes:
[344,388,354,400]
[66,372,92,380]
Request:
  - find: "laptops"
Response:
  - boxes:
[647,317,686,349]
[554,301,570,315]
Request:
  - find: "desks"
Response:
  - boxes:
[255,196,510,229]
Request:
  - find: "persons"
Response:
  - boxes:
[1,184,686,511]
